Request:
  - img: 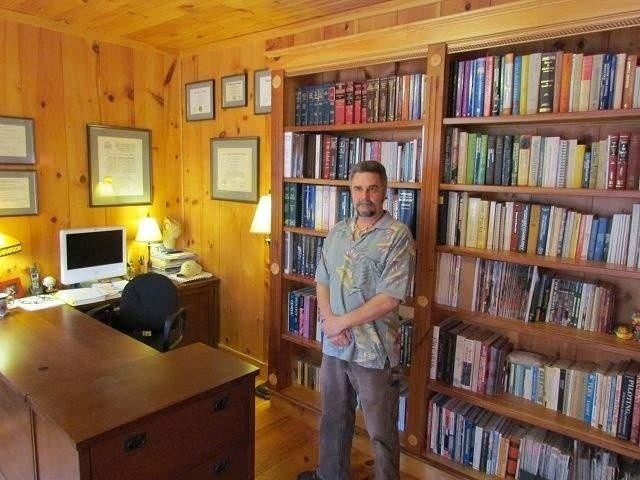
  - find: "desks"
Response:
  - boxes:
[0,276,162,480]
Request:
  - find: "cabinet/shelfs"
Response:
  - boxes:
[265,20,426,461]
[416,0,640,480]
[25,341,259,480]
[76,280,221,348]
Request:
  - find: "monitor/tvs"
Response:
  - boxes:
[60,226,127,286]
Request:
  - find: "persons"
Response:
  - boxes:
[297,161,417,480]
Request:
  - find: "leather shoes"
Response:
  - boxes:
[297,471,321,480]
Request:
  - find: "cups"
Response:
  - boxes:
[0,293,14,316]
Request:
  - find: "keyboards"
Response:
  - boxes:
[58,280,128,306]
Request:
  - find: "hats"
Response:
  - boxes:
[163,216,181,249]
[177,260,202,277]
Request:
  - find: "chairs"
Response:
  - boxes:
[87,272,191,352]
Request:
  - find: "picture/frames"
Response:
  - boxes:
[209,136,259,204]
[85,124,153,207]
[253,68,272,115]
[1,169,40,216]
[0,116,38,165]
[0,277,25,299]
[185,78,214,121]
[220,72,246,108]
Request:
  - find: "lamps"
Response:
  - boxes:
[134,213,164,274]
[249,188,271,402]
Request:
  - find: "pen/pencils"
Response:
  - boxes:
[137,256,146,266]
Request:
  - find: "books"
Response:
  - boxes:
[59,289,108,307]
[284,75,427,431]
[151,252,197,274]
[425,51,640,477]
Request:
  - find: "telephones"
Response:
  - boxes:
[28,262,43,295]
